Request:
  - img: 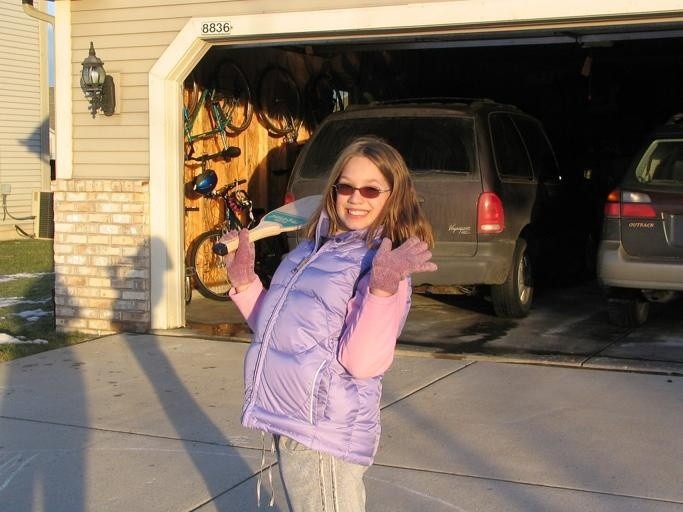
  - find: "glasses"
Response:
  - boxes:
[332,184,392,198]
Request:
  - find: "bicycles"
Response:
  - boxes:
[258,63,345,144]
[180,61,253,159]
[186,180,273,296]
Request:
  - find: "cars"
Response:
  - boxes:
[594,112,681,328]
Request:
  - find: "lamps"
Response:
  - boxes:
[80,41,106,119]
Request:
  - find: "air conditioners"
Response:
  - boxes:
[31,190,55,241]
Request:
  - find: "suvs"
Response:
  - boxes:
[282,100,568,314]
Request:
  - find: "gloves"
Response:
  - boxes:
[369,237,438,294]
[218,228,256,286]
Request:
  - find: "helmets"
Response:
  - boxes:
[193,170,217,193]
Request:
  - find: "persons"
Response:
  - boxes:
[221,135,437,512]
